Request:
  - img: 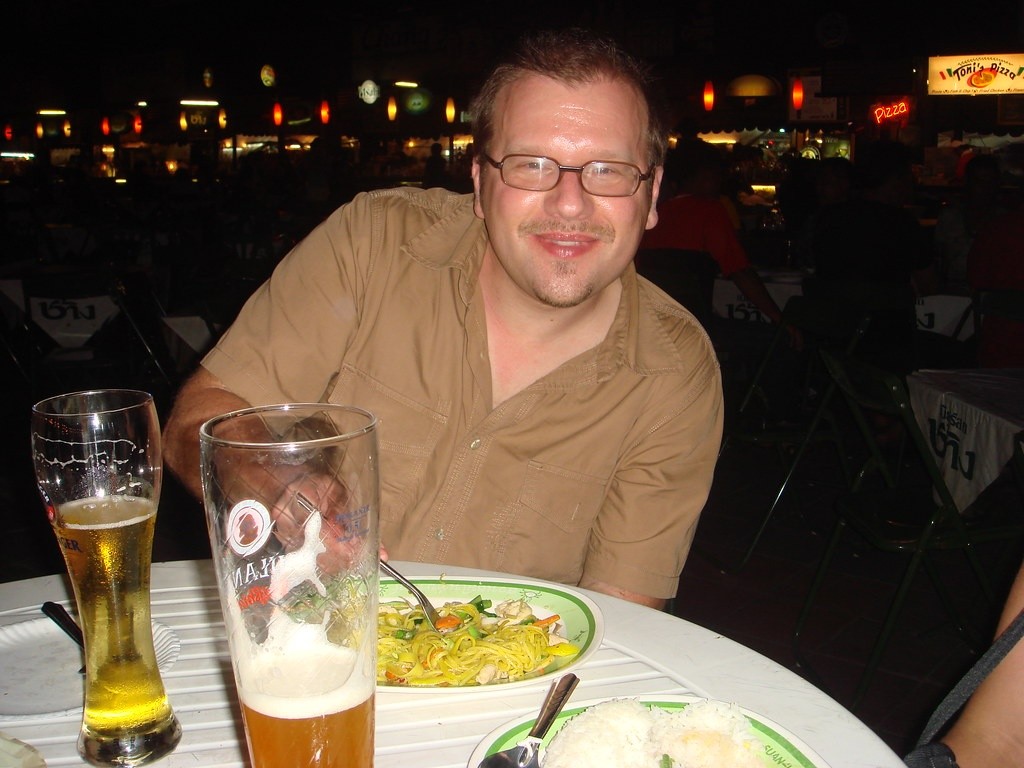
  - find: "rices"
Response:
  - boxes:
[536,696,773,768]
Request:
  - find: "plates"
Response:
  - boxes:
[0,612,181,723]
[292,578,605,694]
[467,695,830,768]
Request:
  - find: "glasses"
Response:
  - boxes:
[481,148,656,198]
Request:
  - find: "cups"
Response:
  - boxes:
[198,403,380,768]
[32,388,182,768]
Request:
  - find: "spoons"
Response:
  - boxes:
[477,674,579,767]
[297,492,447,640]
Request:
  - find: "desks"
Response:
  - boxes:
[0,541,910,767]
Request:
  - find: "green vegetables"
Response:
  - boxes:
[395,594,540,640]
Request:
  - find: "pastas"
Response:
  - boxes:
[371,602,553,685]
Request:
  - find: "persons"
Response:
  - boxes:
[638,129,1023,445]
[159,28,724,612]
[902,557,1024,768]
